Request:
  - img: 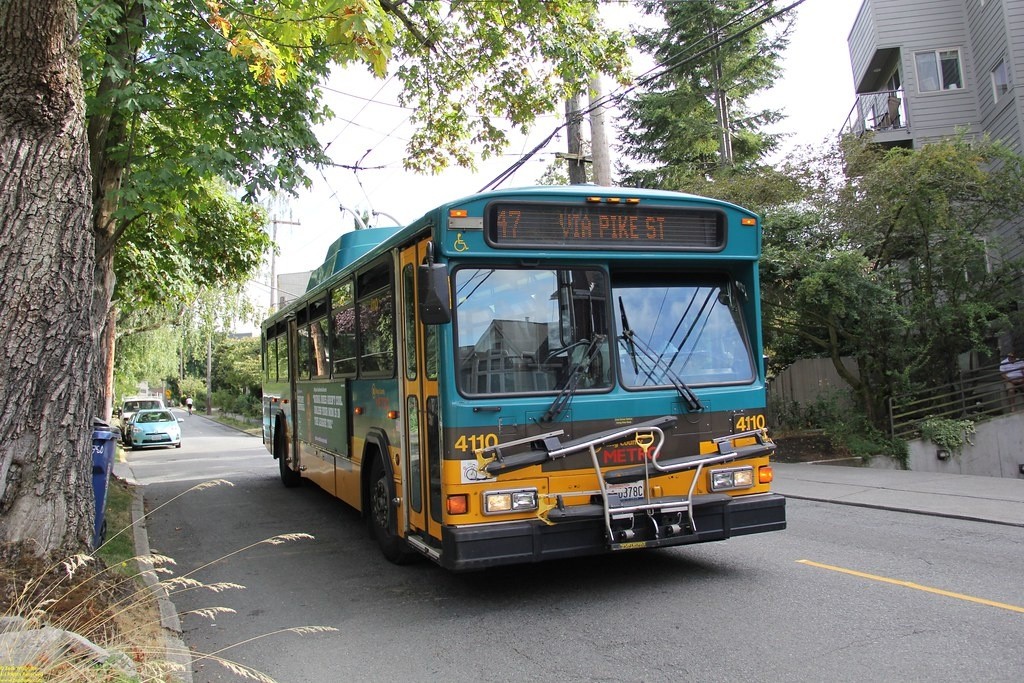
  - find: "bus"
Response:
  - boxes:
[258,182,789,574]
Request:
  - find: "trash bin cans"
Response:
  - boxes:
[91,416,121,546]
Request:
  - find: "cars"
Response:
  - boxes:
[126,409,185,451]
[124,413,137,446]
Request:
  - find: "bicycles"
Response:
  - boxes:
[188,405,192,417]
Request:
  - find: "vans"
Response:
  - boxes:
[118,396,169,443]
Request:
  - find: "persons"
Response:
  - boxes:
[186,396,193,415]
[634,320,679,352]
[116,405,121,419]
[1000,351,1024,411]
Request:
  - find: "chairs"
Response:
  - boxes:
[870,112,900,132]
